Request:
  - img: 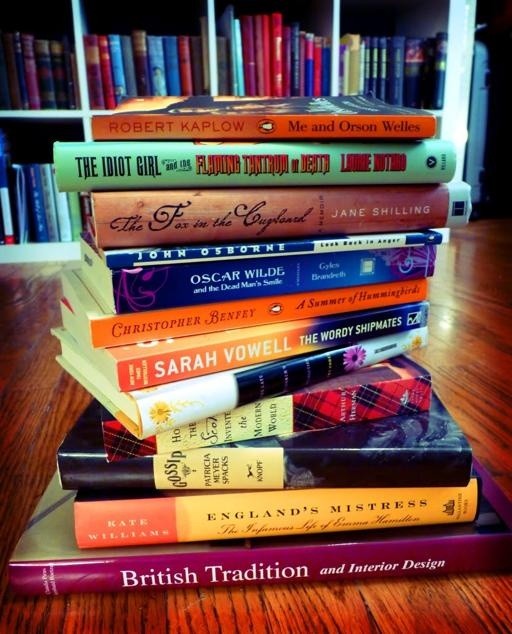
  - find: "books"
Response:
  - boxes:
[7,94,512,597]
[2,14,449,245]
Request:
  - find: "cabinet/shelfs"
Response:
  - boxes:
[0,0,473,265]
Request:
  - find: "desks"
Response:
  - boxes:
[0,215,512,634]
